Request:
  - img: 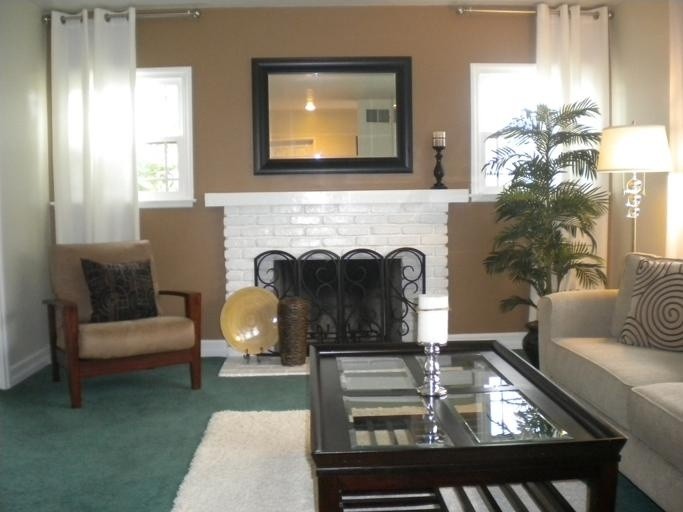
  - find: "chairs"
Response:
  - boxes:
[42,235,203,408]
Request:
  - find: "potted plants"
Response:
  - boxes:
[476,93,614,371]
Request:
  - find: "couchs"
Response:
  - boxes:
[535,251,683,511]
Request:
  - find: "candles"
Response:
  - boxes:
[432,129,446,148]
[416,293,449,344]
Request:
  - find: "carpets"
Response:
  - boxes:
[217,352,314,378]
[170,401,591,511]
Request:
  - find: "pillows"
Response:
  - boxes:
[78,255,159,322]
[619,254,682,353]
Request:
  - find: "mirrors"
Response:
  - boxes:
[250,55,415,175]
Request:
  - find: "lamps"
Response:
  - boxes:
[595,119,676,252]
[302,89,317,109]
